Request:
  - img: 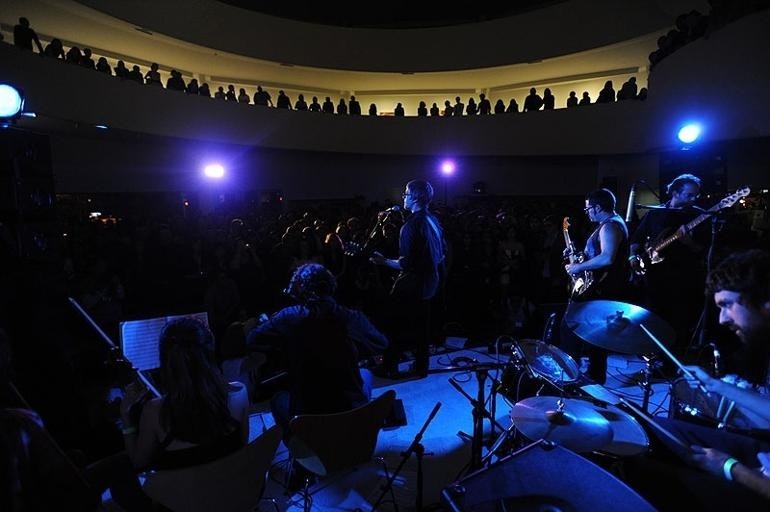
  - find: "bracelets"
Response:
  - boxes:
[723,458,739,480]
[122,427,136,434]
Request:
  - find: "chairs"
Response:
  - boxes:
[287,388,406,476]
[137,422,286,511]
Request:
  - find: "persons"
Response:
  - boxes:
[656,249,767,496]
[0,0,770,116]
[120,316,243,470]
[560,186,628,375]
[372,179,446,378]
[630,175,716,372]
[80,195,585,384]
[246,262,387,455]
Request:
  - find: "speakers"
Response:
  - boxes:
[0,126,67,260]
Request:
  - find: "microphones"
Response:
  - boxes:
[511,341,541,386]
[385,205,400,213]
[626,183,637,223]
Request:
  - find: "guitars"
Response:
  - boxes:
[562,216,598,297]
[629,187,750,277]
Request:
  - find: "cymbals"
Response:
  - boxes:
[512,396,614,452]
[565,300,669,354]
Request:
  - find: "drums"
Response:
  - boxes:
[501,342,582,404]
[668,375,765,435]
[572,397,649,478]
